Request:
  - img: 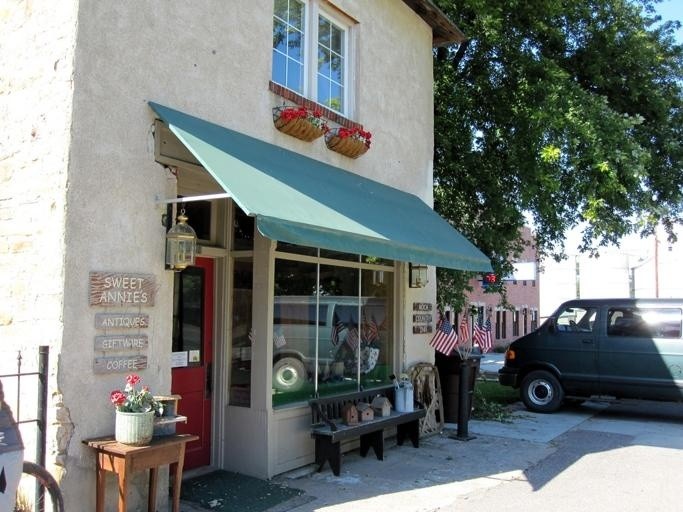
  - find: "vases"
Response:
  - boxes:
[114,408,155,447]
[326,133,367,159]
[272,114,323,142]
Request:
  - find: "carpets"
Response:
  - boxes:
[166,469,317,512]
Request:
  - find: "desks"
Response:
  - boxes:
[81,434,198,512]
[310,385,426,476]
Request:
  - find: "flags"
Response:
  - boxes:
[361,310,368,346]
[344,316,363,351]
[473,312,485,350]
[428,316,459,357]
[366,315,380,346]
[330,310,346,347]
[460,312,470,345]
[477,317,492,354]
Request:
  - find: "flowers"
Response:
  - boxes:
[336,126,371,150]
[110,375,163,416]
[279,104,328,132]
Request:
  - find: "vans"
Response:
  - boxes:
[271,295,388,393]
[497,298,683,414]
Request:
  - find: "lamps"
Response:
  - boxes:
[164,203,198,272]
[408,262,429,289]
